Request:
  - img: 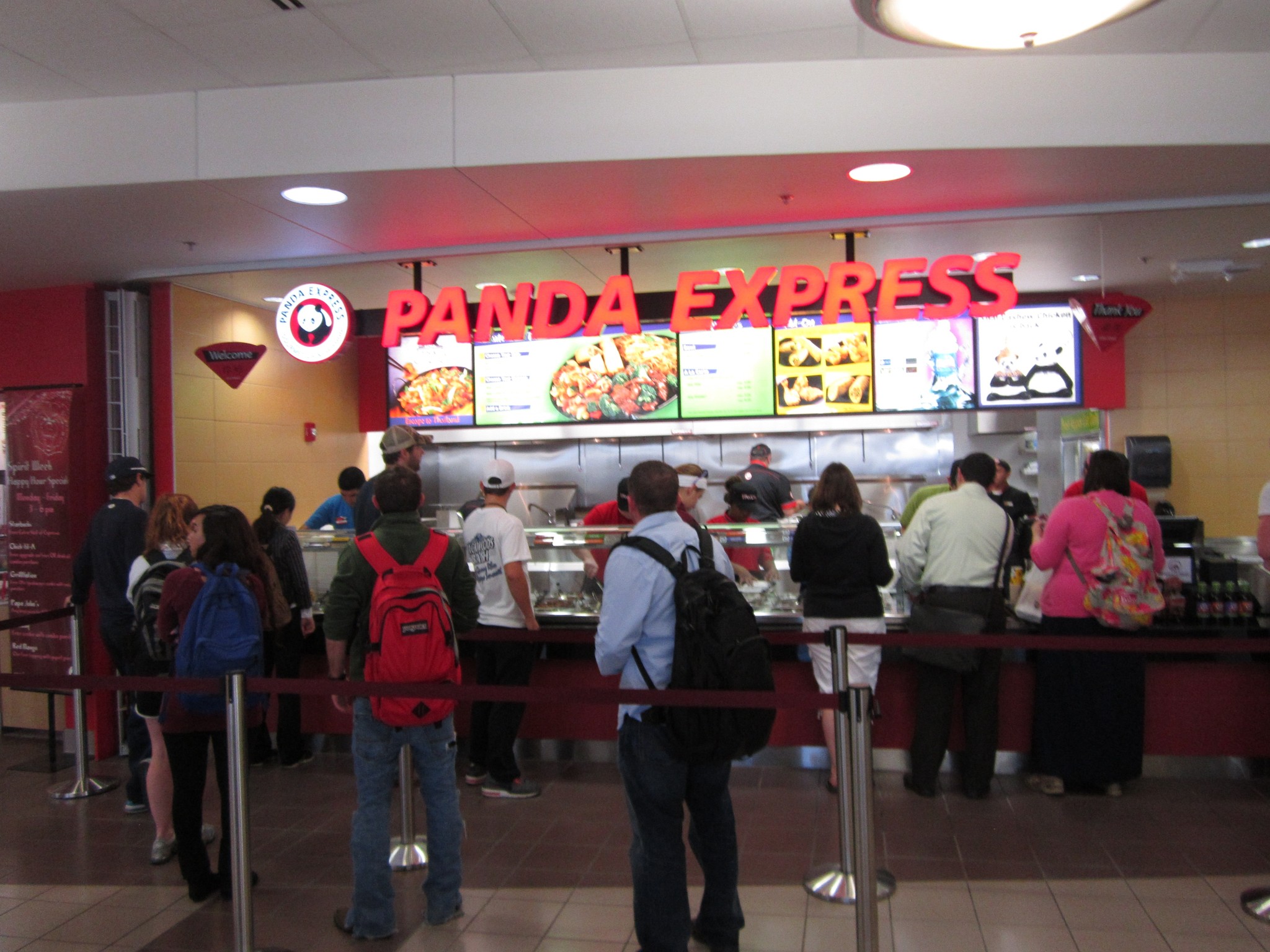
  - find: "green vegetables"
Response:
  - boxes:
[404,373,472,415]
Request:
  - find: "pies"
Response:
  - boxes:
[778,336,823,366]
[827,376,869,403]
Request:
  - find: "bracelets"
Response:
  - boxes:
[329,674,346,680]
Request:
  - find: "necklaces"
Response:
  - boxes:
[482,503,506,511]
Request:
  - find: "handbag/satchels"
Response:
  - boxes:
[902,600,983,670]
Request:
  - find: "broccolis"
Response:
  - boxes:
[588,362,679,418]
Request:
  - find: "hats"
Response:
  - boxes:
[380,424,433,453]
[995,459,1010,471]
[617,478,628,512]
[482,459,515,489]
[104,457,154,484]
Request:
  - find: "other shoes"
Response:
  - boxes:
[1104,780,1124,796]
[334,906,390,940]
[903,772,934,796]
[1023,772,1064,795]
[827,781,838,792]
[446,902,464,919]
[123,793,258,904]
[251,753,274,766]
[958,776,986,799]
[282,751,313,769]
[689,917,740,952]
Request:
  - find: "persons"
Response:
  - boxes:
[324,462,479,941]
[463,458,539,799]
[124,493,214,861]
[787,487,817,563]
[64,456,153,812]
[567,477,636,594]
[736,444,807,522]
[1026,450,1165,798]
[593,460,746,952]
[790,462,893,797]
[157,505,274,905]
[458,490,485,520]
[300,465,367,557]
[354,425,434,535]
[706,475,779,587]
[675,463,709,526]
[245,486,315,769]
[895,452,1034,799]
[1257,483,1270,570]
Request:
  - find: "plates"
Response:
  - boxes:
[393,366,475,416]
[550,333,678,421]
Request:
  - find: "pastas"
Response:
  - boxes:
[614,333,677,372]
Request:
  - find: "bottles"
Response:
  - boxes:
[1197,579,1254,619]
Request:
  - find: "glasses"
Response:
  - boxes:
[694,470,708,485]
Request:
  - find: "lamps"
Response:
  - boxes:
[849,0,1161,49]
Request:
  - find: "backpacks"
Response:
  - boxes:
[1065,492,1166,631]
[174,561,261,710]
[351,529,460,726]
[133,550,192,674]
[606,524,775,760]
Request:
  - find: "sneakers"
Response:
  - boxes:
[481,775,540,798]
[465,763,489,785]
